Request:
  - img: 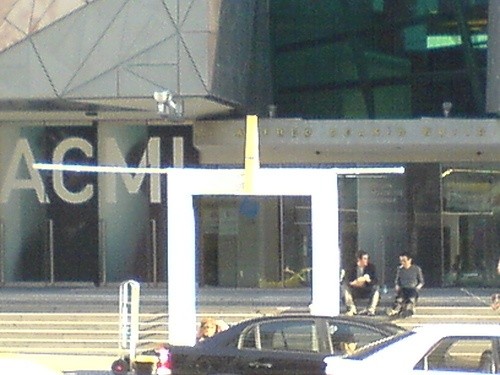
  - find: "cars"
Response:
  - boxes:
[158,316,403,375]
[323,322,499,375]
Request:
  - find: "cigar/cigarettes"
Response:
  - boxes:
[398,265,404,270]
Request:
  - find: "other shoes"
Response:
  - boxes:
[345,305,358,316]
[367,307,375,315]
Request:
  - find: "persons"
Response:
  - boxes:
[335,331,360,356]
[391,251,423,315]
[340,250,381,316]
[437,99,455,119]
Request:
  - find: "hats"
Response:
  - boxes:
[333,327,357,344]
